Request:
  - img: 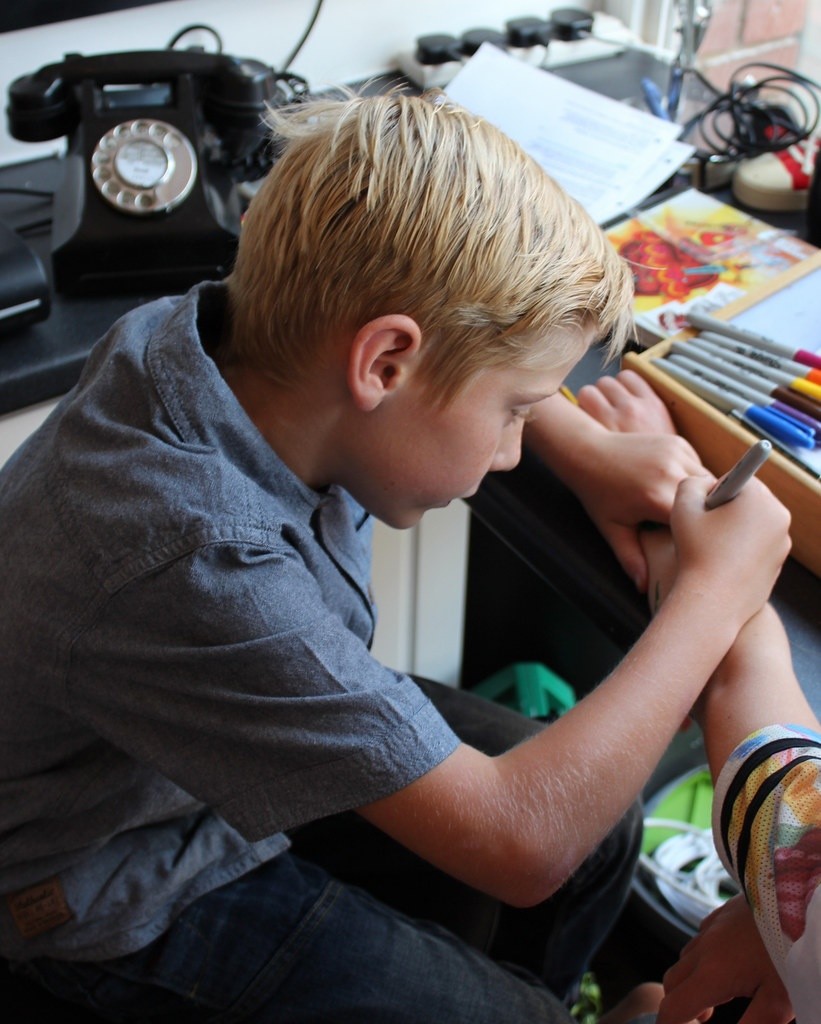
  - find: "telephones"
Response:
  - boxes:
[5,48,277,296]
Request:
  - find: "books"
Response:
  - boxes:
[601,188,821,342]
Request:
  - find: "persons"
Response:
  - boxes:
[580,368,820,1024]
[1,86,795,1024]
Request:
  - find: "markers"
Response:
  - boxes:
[705,438,771,511]
[650,310,821,484]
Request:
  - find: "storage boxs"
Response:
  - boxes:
[629,763,740,939]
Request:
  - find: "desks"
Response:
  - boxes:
[1,21,819,802]
